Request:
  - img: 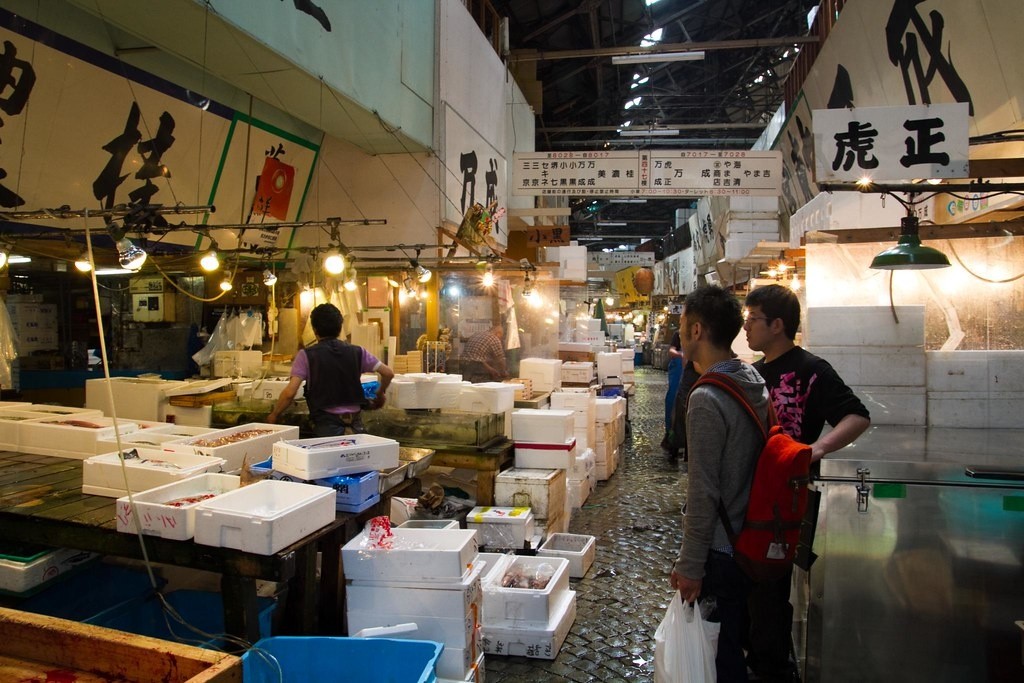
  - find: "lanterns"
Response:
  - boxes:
[636,267,654,296]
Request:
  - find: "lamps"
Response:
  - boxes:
[0,215,533,297]
[192,97,212,109]
[776,251,795,270]
[870,177,950,272]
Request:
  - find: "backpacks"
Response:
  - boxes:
[686,371,812,585]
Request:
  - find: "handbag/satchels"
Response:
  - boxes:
[654,590,720,683]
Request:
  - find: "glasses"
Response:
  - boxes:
[743,316,774,322]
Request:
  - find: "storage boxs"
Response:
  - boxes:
[0,276,635,683]
[801,304,1024,488]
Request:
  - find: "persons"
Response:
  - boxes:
[671,286,776,683]
[266,303,395,437]
[460,325,509,380]
[744,285,872,476]
[665,331,704,463]
